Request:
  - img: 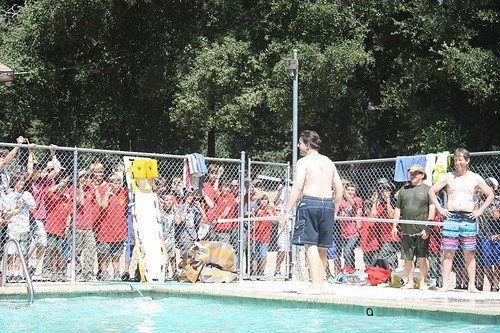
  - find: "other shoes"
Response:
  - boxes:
[122,272,130,280]
[84,274,95,281]
[274,271,282,280]
[8,275,25,283]
[101,270,111,280]
[251,270,263,277]
[113,273,122,281]
[51,273,66,281]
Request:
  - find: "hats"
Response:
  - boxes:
[43,161,66,173]
[77,169,91,179]
[485,177,498,187]
[407,165,427,180]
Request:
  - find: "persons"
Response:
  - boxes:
[278,130,344,295]
[392,163,437,290]
[0,136,500,292]
[428,149,495,293]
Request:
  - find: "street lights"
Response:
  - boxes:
[284,49,305,283]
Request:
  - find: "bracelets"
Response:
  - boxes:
[283,209,290,214]
[56,183,61,190]
[480,205,486,212]
[202,193,207,199]
[425,220,433,229]
